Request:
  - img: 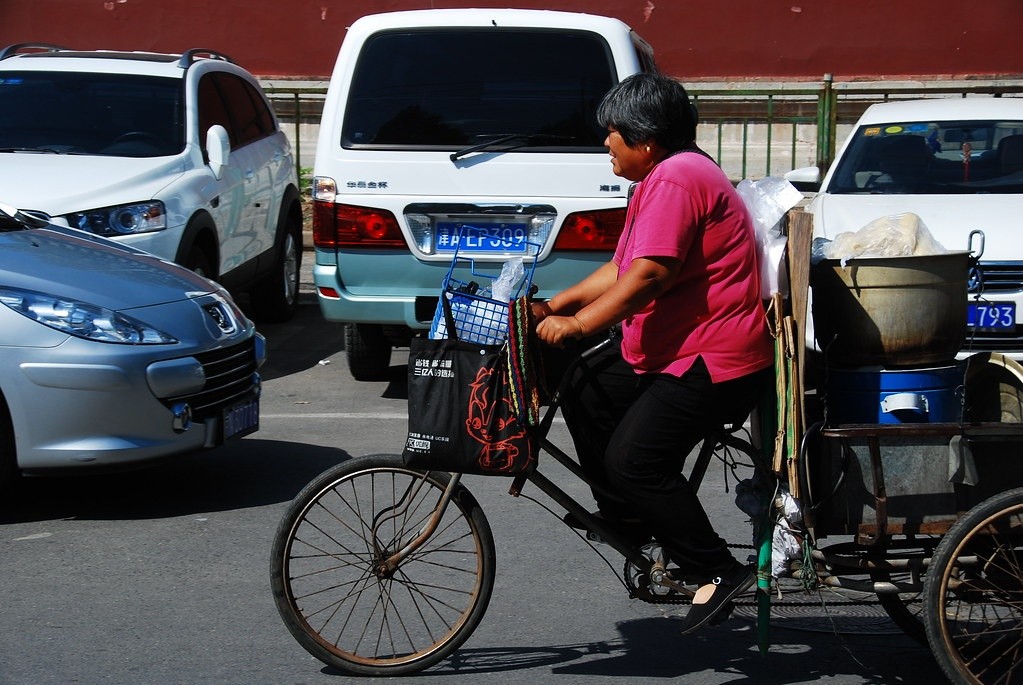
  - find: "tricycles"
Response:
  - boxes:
[269,202,1023,683]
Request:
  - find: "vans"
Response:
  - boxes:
[306,5,658,389]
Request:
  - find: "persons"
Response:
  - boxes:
[529,73,768,635]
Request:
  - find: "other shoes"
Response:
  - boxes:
[680,562,758,637]
[563,511,613,532]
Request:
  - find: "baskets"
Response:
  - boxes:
[428,225,543,346]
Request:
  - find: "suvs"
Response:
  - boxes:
[1,44,301,324]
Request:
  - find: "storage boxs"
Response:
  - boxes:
[429,226,541,346]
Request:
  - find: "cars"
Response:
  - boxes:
[787,98,1020,340]
[0,208,268,492]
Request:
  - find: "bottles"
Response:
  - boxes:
[447,280,479,308]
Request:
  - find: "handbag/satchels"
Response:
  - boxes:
[402,279,539,478]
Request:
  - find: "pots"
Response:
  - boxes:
[811,230,985,365]
